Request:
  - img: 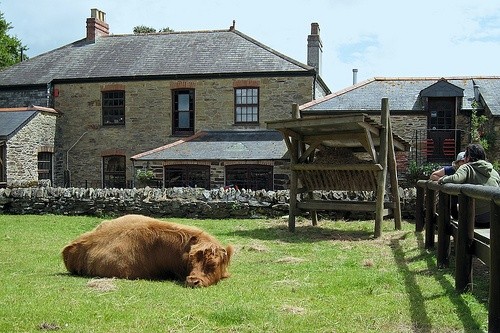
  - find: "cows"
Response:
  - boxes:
[62,213,234,288]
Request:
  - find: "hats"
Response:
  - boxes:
[451,152,466,166]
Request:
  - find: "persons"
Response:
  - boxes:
[438,143,500,229]
[430,151,465,182]
